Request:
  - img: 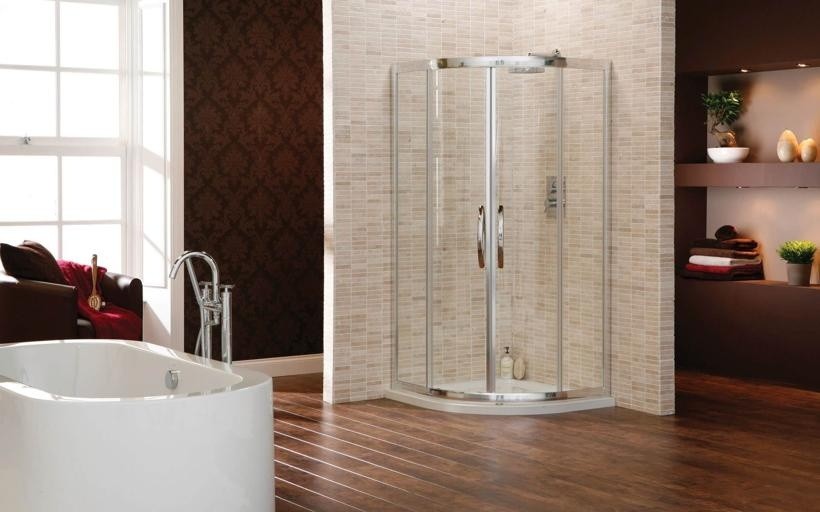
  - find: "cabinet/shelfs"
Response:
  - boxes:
[674,0,820,387]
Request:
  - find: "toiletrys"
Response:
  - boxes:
[500,346,514,379]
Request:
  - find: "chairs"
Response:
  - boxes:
[1,260,143,342]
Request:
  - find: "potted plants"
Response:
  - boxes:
[776,238,817,286]
[699,88,750,163]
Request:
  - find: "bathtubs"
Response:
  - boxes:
[0,337,278,512]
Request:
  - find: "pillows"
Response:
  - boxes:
[1,239,68,284]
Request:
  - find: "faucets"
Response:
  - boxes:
[169,248,222,326]
